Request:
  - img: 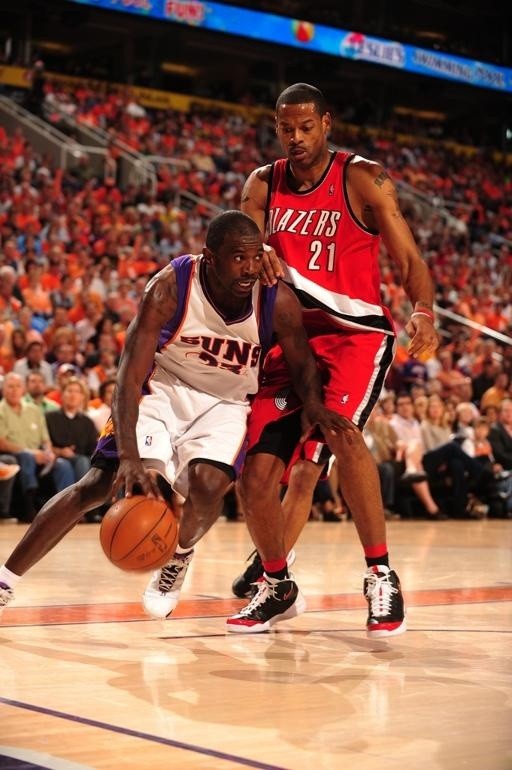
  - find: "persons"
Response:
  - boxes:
[0,58,512,526]
[0,210,357,617]
[231,427,330,599]
[226,82,436,639]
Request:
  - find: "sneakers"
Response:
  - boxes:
[225,574,307,633]
[141,544,196,620]
[360,565,406,639]
[230,542,296,597]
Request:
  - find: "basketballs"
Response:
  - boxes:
[100,495,178,573]
